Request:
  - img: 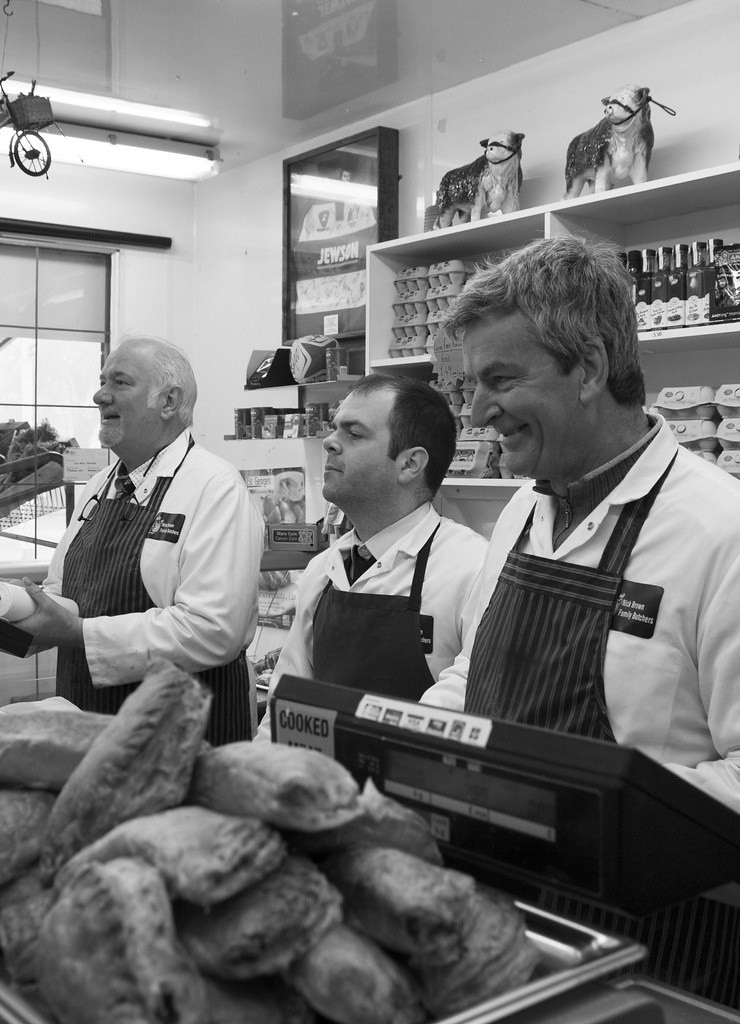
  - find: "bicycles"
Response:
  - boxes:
[0,71,52,175]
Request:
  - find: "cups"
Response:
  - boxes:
[326,348,349,381]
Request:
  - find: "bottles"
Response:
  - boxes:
[616,239,724,333]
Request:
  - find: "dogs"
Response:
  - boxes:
[563,81,656,204]
[430,129,527,229]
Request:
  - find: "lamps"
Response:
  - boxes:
[0,112,223,182]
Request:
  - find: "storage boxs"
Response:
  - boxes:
[268,522,332,553]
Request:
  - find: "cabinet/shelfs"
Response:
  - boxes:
[221,379,364,554]
[364,160,740,499]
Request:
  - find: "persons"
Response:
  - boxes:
[8,337,266,747]
[419,237,740,817]
[253,375,490,746]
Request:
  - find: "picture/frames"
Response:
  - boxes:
[280,126,401,347]
[279,0,401,120]
